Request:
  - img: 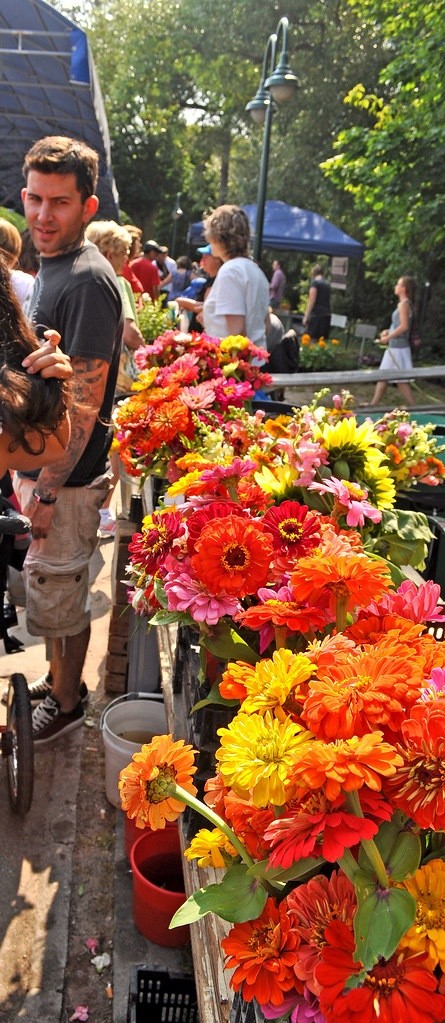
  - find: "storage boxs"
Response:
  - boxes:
[229,980,275,1023]
[172,647,217,845]
[126,965,200,1023]
[177,618,241,782]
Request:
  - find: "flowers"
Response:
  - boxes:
[98,291,445,1023]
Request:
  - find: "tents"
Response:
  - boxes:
[187,199,364,349]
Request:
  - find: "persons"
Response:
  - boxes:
[303,264,331,340]
[201,204,284,374]
[129,240,165,302]
[121,224,144,294]
[153,246,199,299]
[370,276,415,406]
[176,244,224,334]
[269,259,286,308]
[0,217,73,477]
[3,135,125,744]
[86,220,146,535]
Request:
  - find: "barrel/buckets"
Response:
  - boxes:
[100,692,167,810]
[122,809,190,949]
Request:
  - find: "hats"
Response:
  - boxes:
[143,240,165,254]
[197,244,212,254]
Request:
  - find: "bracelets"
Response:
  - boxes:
[33,489,57,504]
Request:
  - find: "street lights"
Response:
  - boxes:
[167,191,183,257]
[244,18,299,269]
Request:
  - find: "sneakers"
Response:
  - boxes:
[32,694,86,744]
[3,670,89,705]
